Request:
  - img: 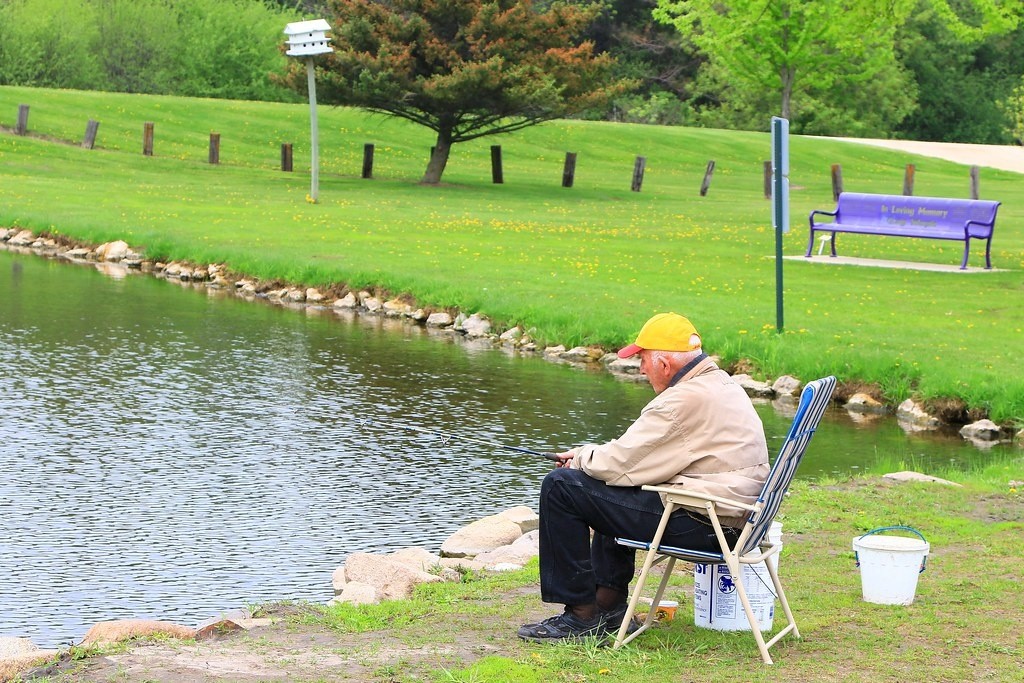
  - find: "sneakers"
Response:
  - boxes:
[516,604,639,643]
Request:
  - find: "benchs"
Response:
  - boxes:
[803,191,1001,272]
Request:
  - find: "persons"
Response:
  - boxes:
[518,312,771,644]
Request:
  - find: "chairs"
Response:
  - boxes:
[607,374,837,664]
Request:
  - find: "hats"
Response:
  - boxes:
[618,312,702,359]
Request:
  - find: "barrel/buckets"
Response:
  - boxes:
[852,526,929,605]
[695,521,783,631]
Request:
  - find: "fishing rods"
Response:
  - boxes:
[293,404,566,466]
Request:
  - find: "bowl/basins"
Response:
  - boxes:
[650,601,679,622]
